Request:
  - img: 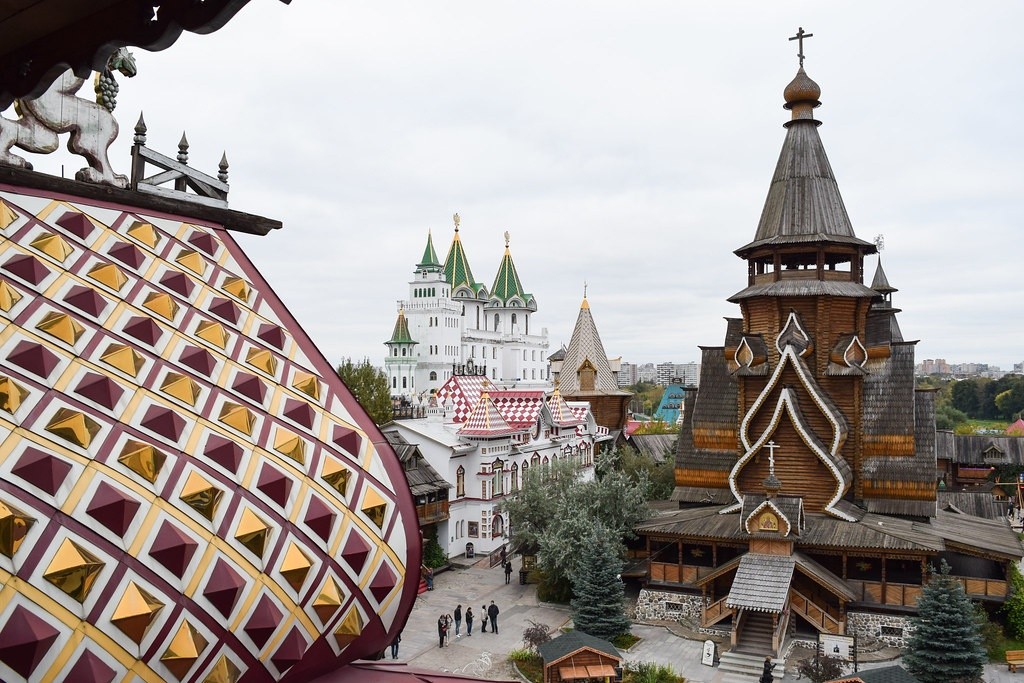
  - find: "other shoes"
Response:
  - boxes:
[467,633,471,636]
[506,583,507,584]
[439,645,443,648]
[508,581,509,583]
[491,631,494,633]
[446,642,449,646]
[482,630,487,632]
[395,655,398,659]
[458,633,463,635]
[456,635,460,638]
[496,631,498,634]
[393,655,394,659]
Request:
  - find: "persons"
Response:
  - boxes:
[500,545,513,585]
[438,600,499,648]
[390,635,402,659]
[759,656,777,683]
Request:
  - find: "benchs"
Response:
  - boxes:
[1005,650,1024,673]
